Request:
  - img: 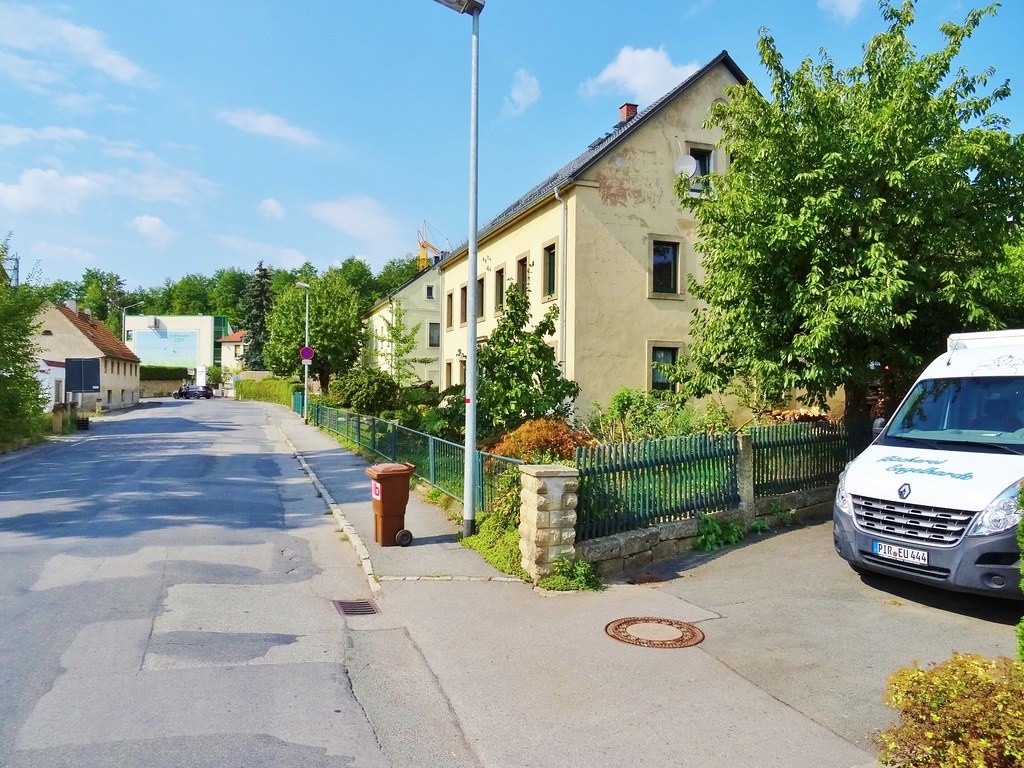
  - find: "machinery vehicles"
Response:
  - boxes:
[416,221,453,271]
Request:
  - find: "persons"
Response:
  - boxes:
[182,383,186,399]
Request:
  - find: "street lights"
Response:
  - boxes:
[295,282,314,425]
[434,0,484,539]
[122,301,145,345]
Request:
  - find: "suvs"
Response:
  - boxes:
[172,386,213,400]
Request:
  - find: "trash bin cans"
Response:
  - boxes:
[51,400,79,434]
[363,461,415,548]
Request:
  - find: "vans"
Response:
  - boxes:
[833,329,1024,608]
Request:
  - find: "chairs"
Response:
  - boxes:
[966,399,1024,433]
